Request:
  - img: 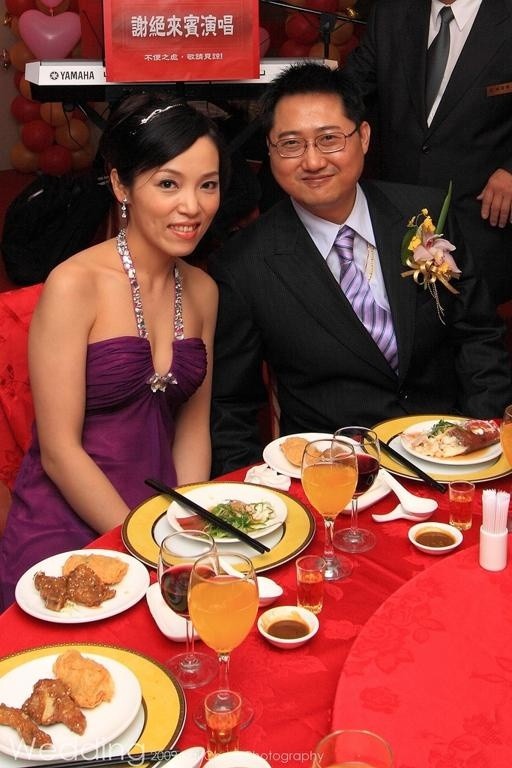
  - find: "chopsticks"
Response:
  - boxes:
[351,422,446,493]
[20,749,180,768]
[144,479,271,554]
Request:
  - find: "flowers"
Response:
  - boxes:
[397,177,462,327]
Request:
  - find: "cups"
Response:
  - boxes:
[449,481,475,531]
[296,554,323,615]
[479,525,508,572]
[204,690,241,759]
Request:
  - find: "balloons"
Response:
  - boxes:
[260,1,366,59]
[5,1,97,176]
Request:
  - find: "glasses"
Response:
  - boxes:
[267,125,360,158]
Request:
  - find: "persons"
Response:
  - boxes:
[344,0,511,304]
[211,58,511,476]
[0,92,233,615]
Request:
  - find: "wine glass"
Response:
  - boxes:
[301,426,381,581]
[157,530,259,734]
[499,404,512,468]
[312,730,394,768]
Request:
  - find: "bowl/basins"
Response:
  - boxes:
[257,605,320,650]
[407,521,464,555]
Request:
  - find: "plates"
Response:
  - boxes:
[121,481,316,576]
[363,414,512,484]
[14,548,150,624]
[203,750,271,768]
[0,641,187,768]
[262,432,357,479]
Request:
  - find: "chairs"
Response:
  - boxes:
[2,280,44,542]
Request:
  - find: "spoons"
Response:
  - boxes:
[160,746,206,768]
[371,469,438,523]
[218,557,283,608]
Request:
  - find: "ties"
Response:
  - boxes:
[426,6,455,120]
[335,225,399,376]
